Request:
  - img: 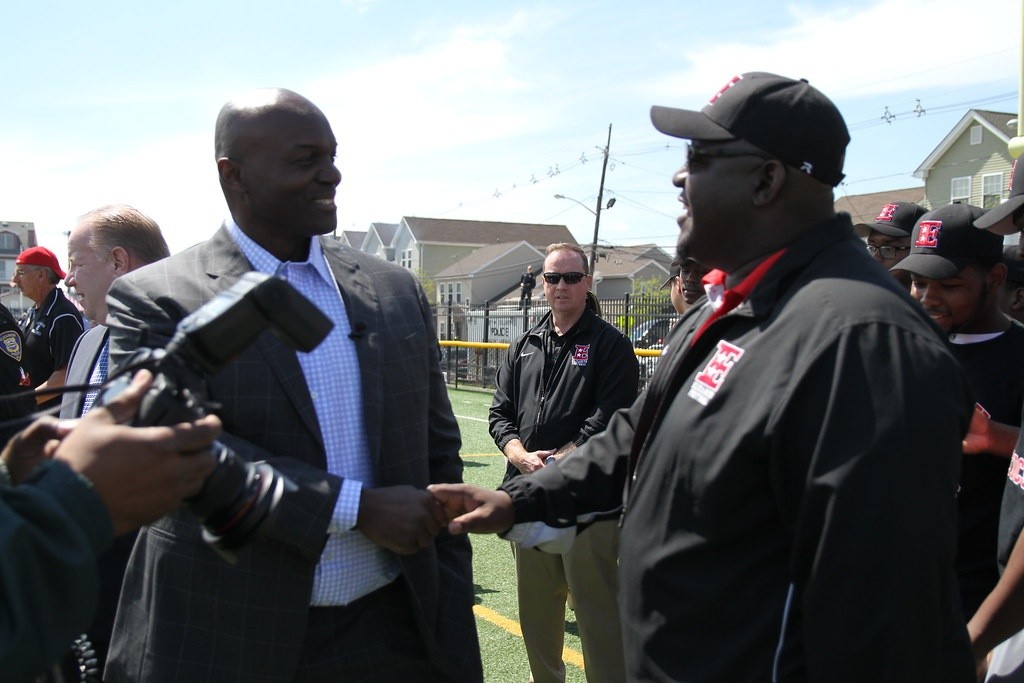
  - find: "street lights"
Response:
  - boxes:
[553,122,617,287]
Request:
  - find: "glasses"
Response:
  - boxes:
[14,268,46,277]
[686,142,790,175]
[866,244,913,259]
[543,271,591,284]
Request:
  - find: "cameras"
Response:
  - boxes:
[86,269,337,563]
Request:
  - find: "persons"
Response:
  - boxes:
[107,90,486,683]
[427,72,977,683]
[488,241,625,683]
[60,204,169,420]
[860,154,1024,683]
[0,245,83,444]
[517,266,536,310]
[0,369,222,683]
[659,256,711,313]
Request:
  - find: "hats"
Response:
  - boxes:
[973,151,1024,235]
[16,246,66,279]
[659,256,681,292]
[649,74,851,185]
[853,201,931,238]
[888,201,1004,280]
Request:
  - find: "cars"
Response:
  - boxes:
[438,345,467,381]
[629,316,678,387]
